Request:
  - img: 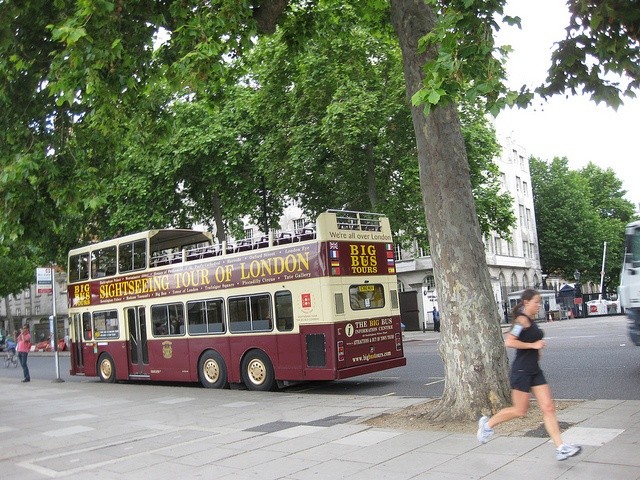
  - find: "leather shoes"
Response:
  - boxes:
[21,377,30,382]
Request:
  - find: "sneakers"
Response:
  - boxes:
[477,417,494,444]
[556,443,580,461]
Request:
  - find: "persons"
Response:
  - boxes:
[5,330,15,361]
[475,287,583,464]
[432,306,440,333]
[16,323,32,383]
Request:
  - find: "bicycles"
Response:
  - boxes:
[5,350,18,368]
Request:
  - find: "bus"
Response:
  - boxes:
[67,210,406,390]
